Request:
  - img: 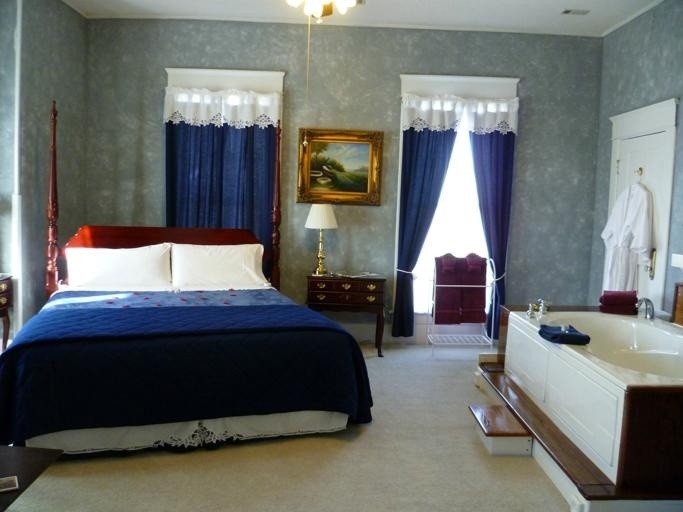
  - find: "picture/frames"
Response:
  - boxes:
[295,126,385,208]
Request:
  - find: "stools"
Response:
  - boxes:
[465,402,534,458]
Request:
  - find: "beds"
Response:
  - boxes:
[0,222,358,463]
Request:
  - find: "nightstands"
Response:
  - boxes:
[307,276,386,360]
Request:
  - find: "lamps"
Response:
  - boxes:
[302,202,339,279]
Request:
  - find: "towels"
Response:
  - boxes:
[536,323,592,346]
[599,290,640,315]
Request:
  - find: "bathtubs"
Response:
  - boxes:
[504,310,683,486]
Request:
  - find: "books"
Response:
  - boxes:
[329,269,366,279]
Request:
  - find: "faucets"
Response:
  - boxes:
[635,297,654,319]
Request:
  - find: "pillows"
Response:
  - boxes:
[64,242,276,294]
